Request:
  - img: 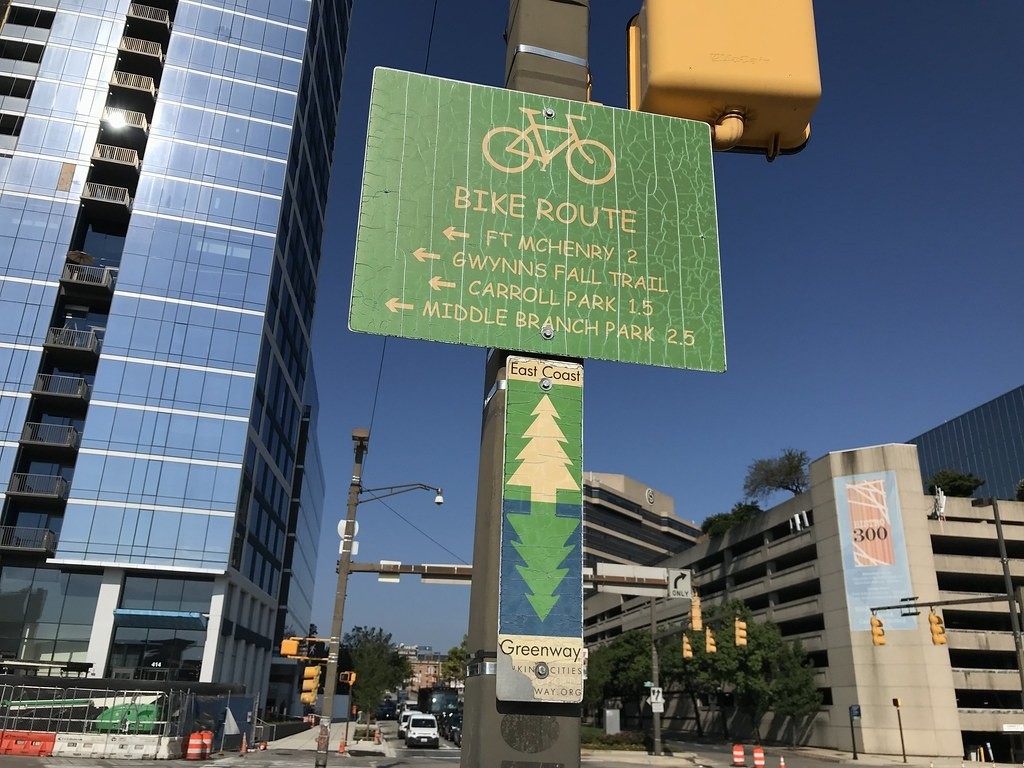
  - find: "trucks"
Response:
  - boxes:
[419,686,460,726]
[398,690,408,701]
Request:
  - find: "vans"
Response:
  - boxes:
[397,711,422,739]
[404,714,440,747]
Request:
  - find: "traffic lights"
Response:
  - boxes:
[928,612,948,644]
[735,617,746,646]
[307,641,325,657]
[688,595,702,632]
[893,698,901,707]
[683,632,693,658]
[300,665,321,704]
[871,614,886,646]
[339,671,356,684]
[706,627,717,652]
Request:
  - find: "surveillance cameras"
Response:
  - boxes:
[434,496,443,505]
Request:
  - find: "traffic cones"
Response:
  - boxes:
[239,731,247,755]
[339,736,346,753]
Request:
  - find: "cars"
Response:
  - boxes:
[441,711,462,748]
[378,700,401,720]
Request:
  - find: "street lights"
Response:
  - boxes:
[314,482,444,768]
[971,497,1024,688]
